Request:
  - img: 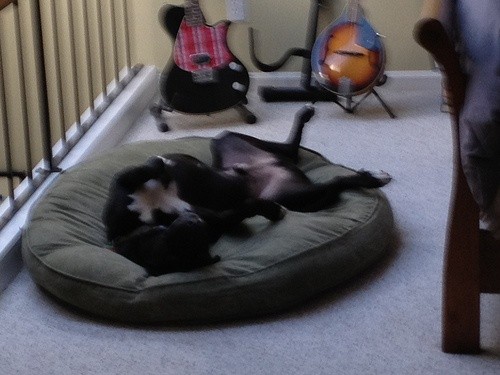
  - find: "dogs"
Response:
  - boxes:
[101,105,391,273]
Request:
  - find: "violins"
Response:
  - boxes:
[311,0,386,94]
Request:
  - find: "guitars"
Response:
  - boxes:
[155,0,251,114]
